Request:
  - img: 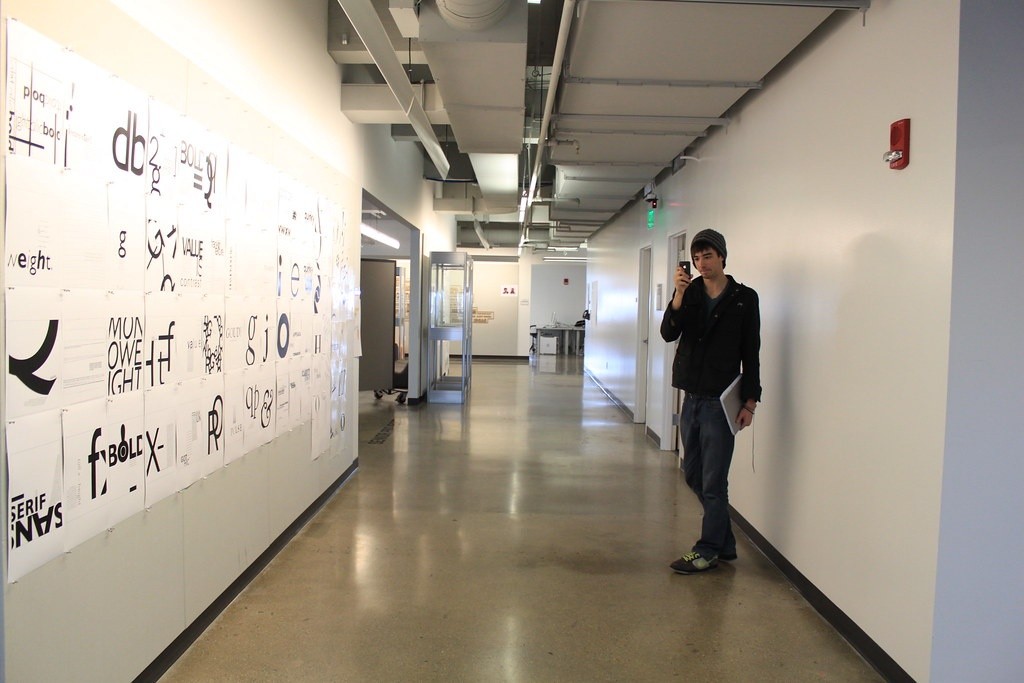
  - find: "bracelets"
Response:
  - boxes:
[743,404,755,415]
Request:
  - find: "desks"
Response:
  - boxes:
[531,326,585,357]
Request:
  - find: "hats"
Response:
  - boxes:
[690,229,727,269]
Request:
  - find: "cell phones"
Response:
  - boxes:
[679,261,690,284]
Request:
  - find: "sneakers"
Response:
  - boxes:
[719,554,737,560]
[669,552,718,574]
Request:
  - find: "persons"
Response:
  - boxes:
[658,227,763,576]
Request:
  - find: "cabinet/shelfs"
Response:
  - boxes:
[427,251,473,404]
